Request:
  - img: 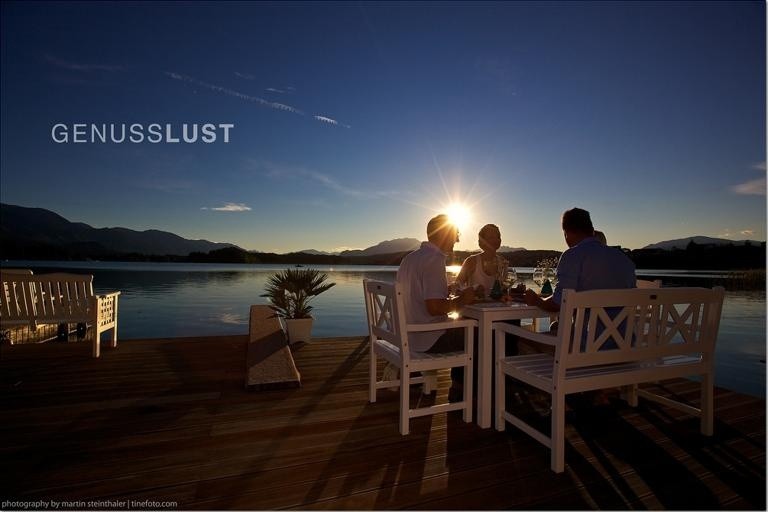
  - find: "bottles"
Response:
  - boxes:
[490,280,502,299]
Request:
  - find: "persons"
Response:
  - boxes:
[518,207,636,425]
[594,230,606,245]
[396,216,478,401]
[455,223,521,389]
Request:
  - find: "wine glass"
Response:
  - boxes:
[501,266,517,306]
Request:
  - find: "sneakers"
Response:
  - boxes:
[590,390,609,406]
[407,371,425,387]
[447,385,463,403]
[564,402,577,417]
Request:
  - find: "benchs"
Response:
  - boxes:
[483,283,727,476]
[0,264,124,361]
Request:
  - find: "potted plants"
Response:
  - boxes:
[253,264,337,345]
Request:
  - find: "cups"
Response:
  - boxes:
[533,268,543,287]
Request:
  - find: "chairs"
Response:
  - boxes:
[616,276,664,402]
[359,278,478,438]
